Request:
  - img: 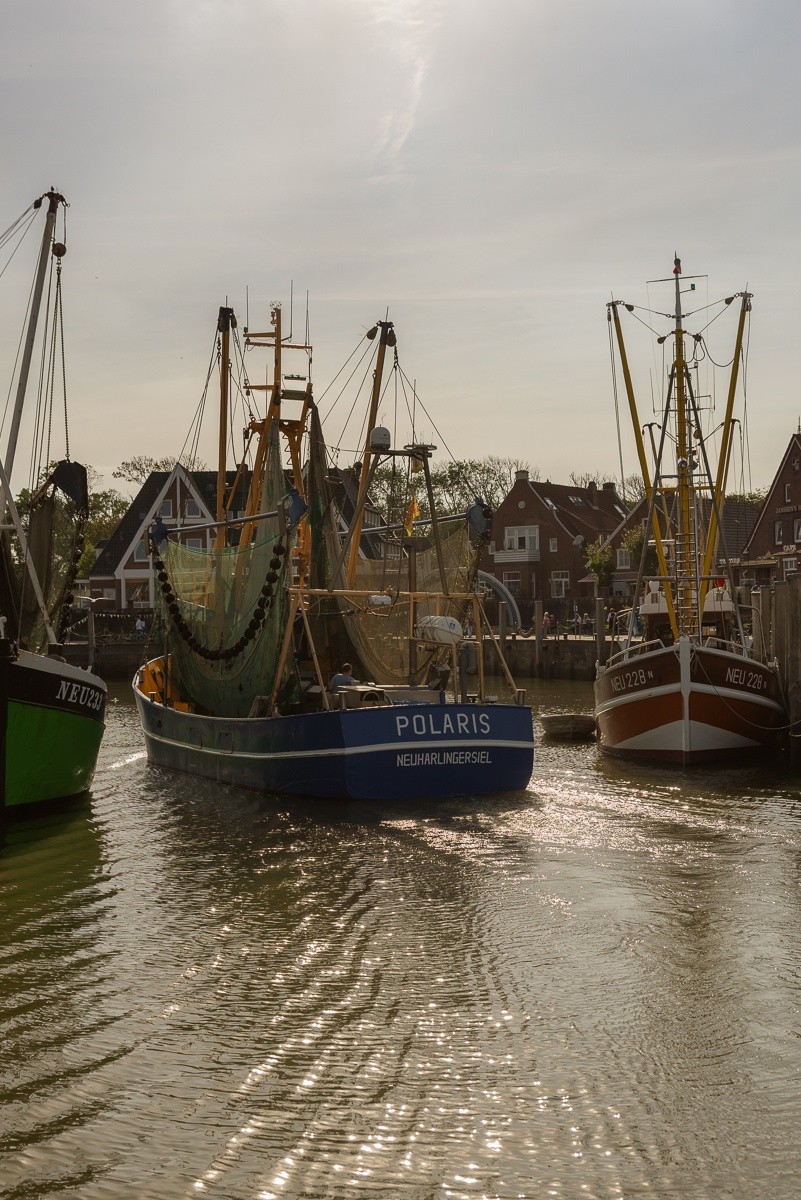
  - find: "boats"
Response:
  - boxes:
[540,712,597,741]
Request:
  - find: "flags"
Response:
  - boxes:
[404,496,420,538]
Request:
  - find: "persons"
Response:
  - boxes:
[467,613,474,638]
[328,663,356,694]
[128,614,146,640]
[520,604,645,639]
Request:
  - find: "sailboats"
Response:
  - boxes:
[128,278,535,802]
[0,185,111,823]
[591,247,793,773]
